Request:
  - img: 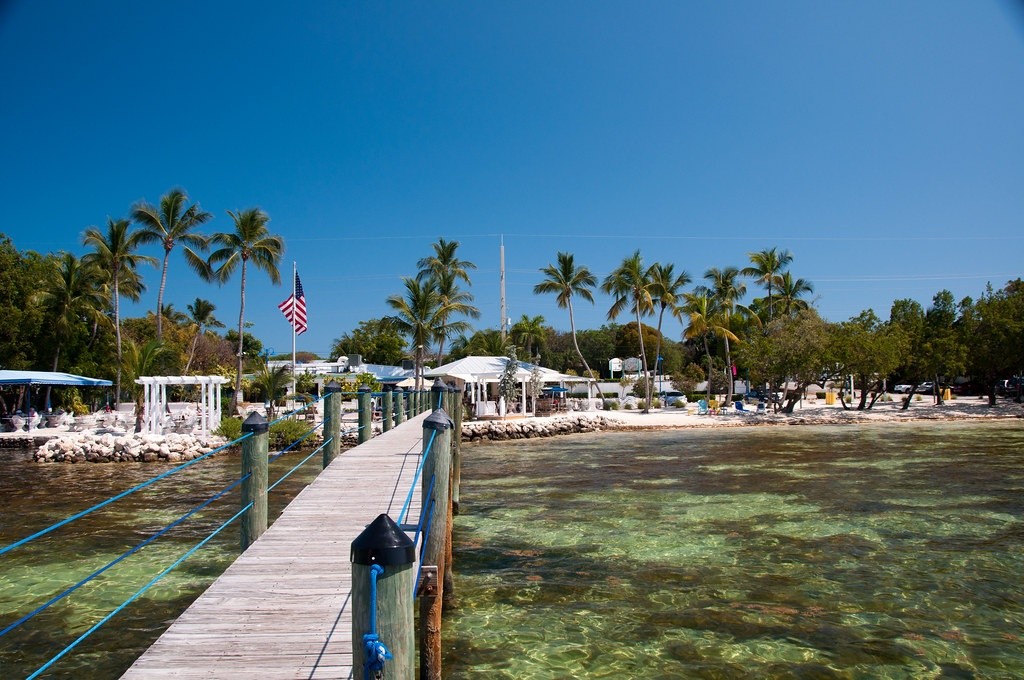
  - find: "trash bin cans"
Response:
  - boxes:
[825,382,836,404]
[939,382,954,400]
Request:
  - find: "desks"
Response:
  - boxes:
[3,414,125,432]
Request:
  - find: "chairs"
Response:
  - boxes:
[12,411,123,431]
[709,400,719,414]
[734,401,749,414]
[698,400,708,415]
[756,402,768,415]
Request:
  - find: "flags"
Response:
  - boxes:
[277,272,309,336]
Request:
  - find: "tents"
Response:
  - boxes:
[0,368,115,433]
